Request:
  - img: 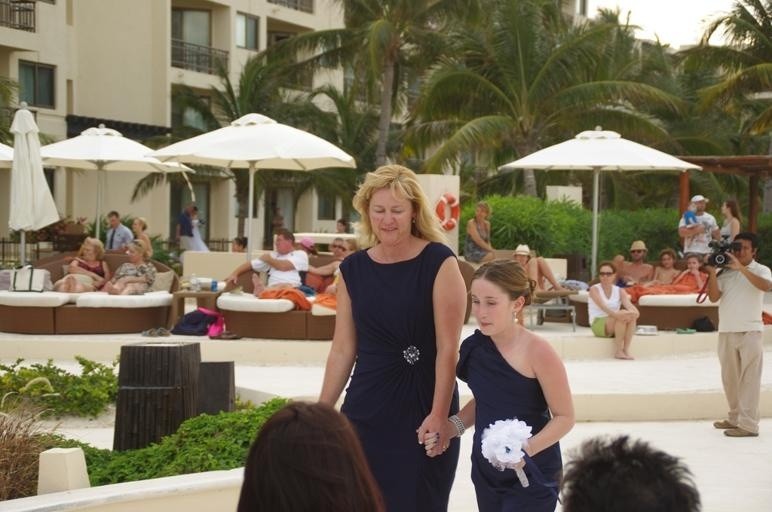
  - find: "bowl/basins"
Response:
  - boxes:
[199,278,211,289]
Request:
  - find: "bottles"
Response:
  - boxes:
[210,272,218,292]
[189,272,199,292]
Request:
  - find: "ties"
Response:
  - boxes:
[107,230,116,250]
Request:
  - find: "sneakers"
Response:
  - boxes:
[724,427,759,438]
[712,420,739,429]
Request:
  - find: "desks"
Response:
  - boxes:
[172,290,221,328]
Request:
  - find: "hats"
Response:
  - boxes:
[690,194,709,203]
[300,237,320,255]
[628,240,649,252]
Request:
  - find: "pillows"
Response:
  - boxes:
[61,264,70,277]
[145,269,175,294]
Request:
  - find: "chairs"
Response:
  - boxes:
[494,249,579,331]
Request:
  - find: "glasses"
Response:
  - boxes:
[599,270,615,277]
[630,250,642,254]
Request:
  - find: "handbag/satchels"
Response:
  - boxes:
[11,263,47,294]
[171,308,219,336]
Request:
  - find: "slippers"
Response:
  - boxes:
[157,327,171,338]
[142,328,157,337]
[207,324,241,342]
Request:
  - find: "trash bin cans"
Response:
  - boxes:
[113,342,200,451]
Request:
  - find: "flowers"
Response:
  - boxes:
[480,418,532,488]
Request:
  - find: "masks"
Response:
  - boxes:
[514,244,530,255]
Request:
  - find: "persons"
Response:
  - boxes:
[233,398,389,512]
[100,240,157,295]
[424,261,575,510]
[106,211,134,250]
[318,165,461,512]
[169,194,749,313]
[130,217,154,260]
[51,237,113,293]
[560,435,703,511]
[586,260,641,362]
[700,233,771,437]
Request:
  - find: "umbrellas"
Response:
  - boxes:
[40,123,195,233]
[148,109,356,244]
[0,143,14,168]
[9,101,61,265]
[496,123,701,281]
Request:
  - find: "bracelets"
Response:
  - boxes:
[448,413,467,438]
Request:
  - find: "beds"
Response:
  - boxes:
[569,261,719,331]
[0,249,184,335]
[215,253,343,340]
